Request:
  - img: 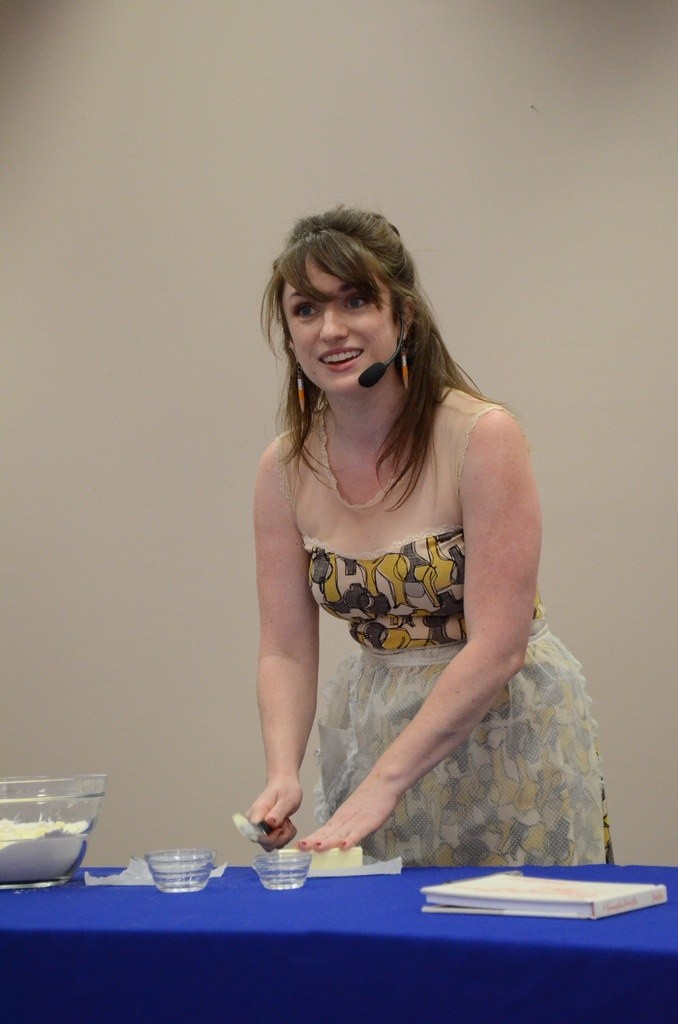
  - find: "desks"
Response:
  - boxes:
[0,864,678,1024]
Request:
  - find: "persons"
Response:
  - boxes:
[240,213,614,869]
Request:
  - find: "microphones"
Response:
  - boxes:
[358,299,404,388]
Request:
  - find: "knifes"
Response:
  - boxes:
[232,813,272,841]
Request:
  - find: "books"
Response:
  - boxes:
[420,872,668,921]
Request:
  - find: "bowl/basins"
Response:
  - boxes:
[250,853,312,890]
[144,848,217,893]
[0,774,108,889]
